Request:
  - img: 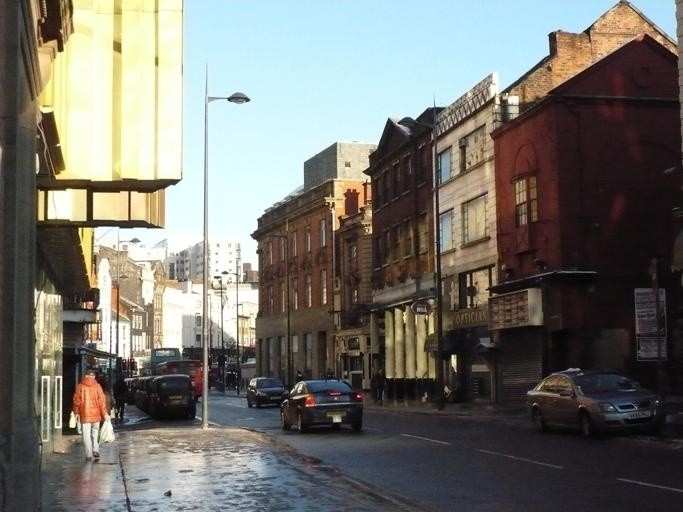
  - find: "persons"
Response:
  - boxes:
[325,368,333,378]
[295,369,304,382]
[71,369,129,462]
[370,368,387,405]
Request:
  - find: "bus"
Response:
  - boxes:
[138,346,254,401]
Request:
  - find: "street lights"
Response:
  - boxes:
[209,274,225,352]
[191,63,257,436]
[263,231,294,387]
[115,237,146,355]
[222,270,242,356]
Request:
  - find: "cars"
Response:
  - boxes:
[244,375,286,408]
[275,376,367,433]
[523,364,665,437]
[123,375,200,419]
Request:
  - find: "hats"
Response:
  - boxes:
[84,370,95,375]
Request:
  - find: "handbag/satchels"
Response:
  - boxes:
[68,410,75,429]
[76,415,82,435]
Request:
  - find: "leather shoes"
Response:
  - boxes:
[86,456,92,461]
[93,451,100,457]
